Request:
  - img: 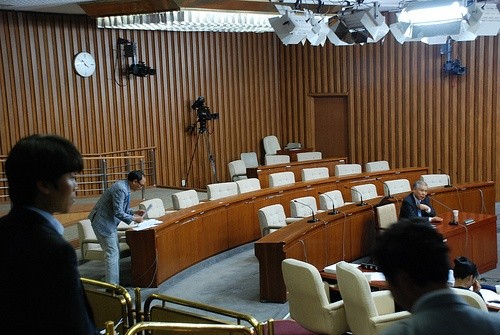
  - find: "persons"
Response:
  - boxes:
[453,256,485,302]
[87,170,146,295]
[0,135,95,335]
[399,181,443,222]
[372,218,500,335]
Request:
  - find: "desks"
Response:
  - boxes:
[431,212,497,276]
[126,167,429,288]
[277,147,312,162]
[253,181,495,304]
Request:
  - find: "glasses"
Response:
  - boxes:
[136,181,145,188]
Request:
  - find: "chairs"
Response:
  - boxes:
[77,135,500,335]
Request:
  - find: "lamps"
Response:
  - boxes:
[78,1,352,34]
[269,0,500,48]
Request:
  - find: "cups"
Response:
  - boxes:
[453,210,459,222]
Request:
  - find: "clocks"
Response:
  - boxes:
[74,52,96,77]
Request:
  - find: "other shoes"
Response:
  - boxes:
[106,288,123,295]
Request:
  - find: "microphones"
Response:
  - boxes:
[318,190,340,215]
[376,177,394,198]
[294,199,320,223]
[344,186,368,206]
[438,168,452,187]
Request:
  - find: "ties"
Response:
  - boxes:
[417,200,422,217]
